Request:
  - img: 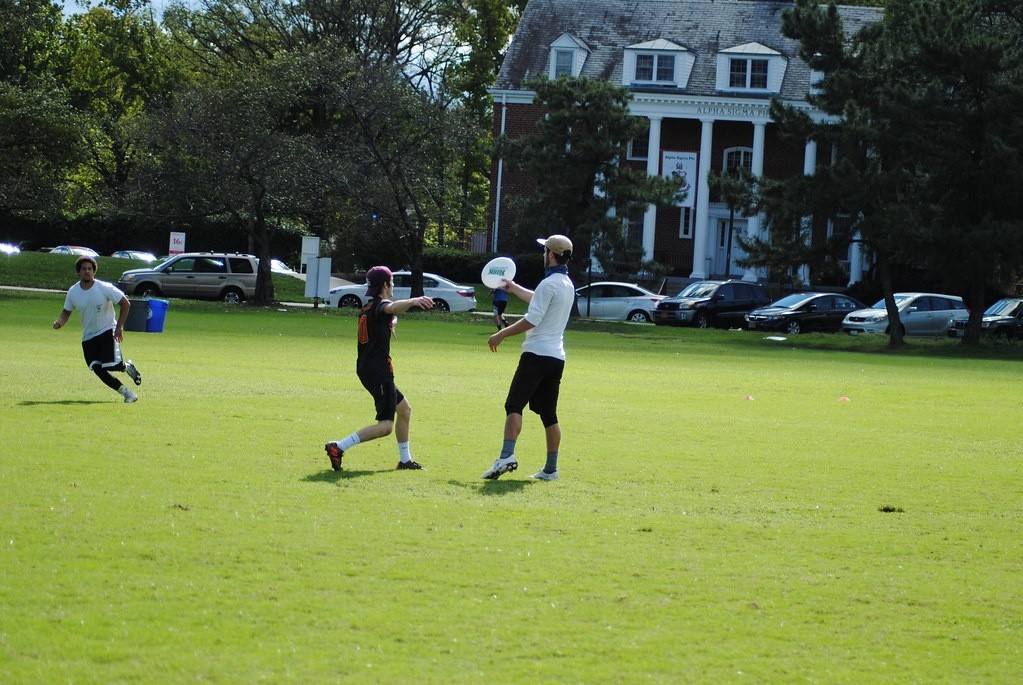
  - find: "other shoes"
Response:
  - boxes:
[124,392,138,403]
[126,359,141,386]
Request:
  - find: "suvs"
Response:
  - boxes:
[650,279,774,329]
[116,250,259,306]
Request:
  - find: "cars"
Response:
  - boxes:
[37,247,54,254]
[324,270,478,313]
[945,297,1023,340]
[573,282,672,323]
[157,252,299,280]
[49,245,100,257]
[841,292,973,338]
[745,291,872,336]
[112,251,157,263]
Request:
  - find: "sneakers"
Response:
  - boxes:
[397,460,425,470]
[526,467,559,481]
[325,441,344,471]
[481,454,518,480]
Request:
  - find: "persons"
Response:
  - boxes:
[490,289,509,332]
[488,235,575,482]
[53,255,142,403]
[325,264,434,470]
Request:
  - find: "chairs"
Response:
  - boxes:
[191,259,204,272]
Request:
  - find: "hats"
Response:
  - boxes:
[365,266,393,296]
[537,235,572,256]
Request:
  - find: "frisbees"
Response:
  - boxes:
[481,257,517,289]
